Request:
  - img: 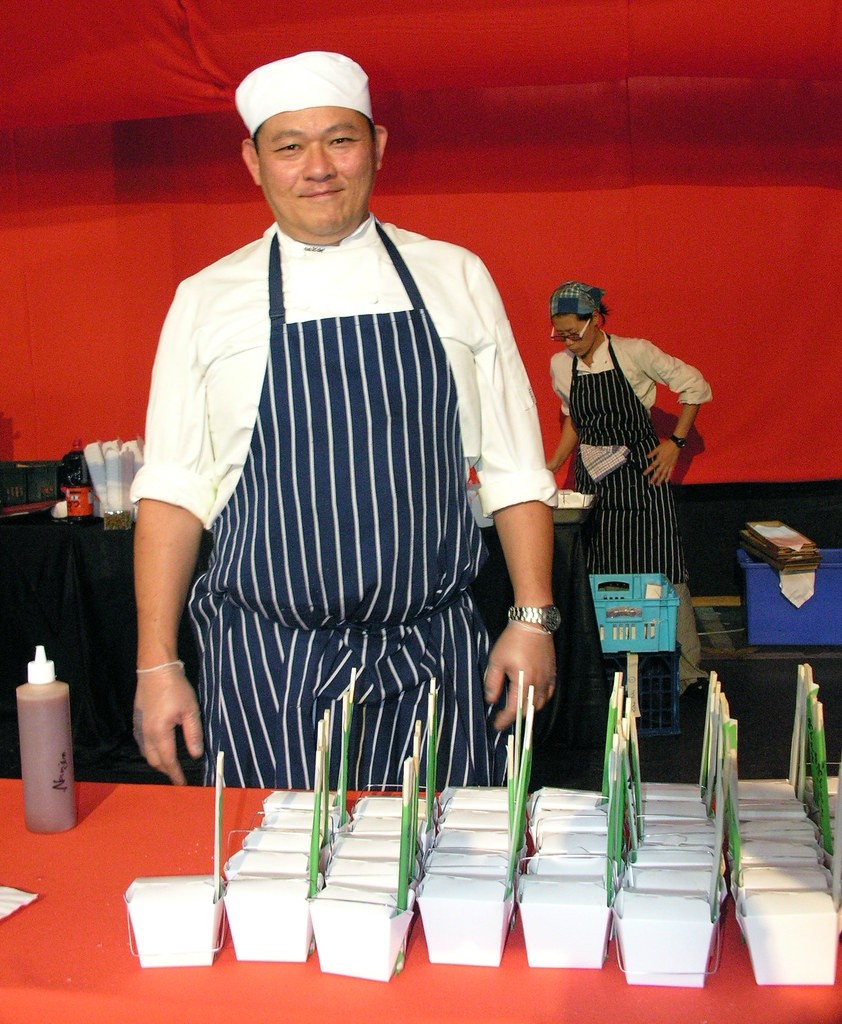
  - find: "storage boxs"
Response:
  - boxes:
[0,459,64,508]
[590,573,681,652]
[603,640,684,736]
[735,549,842,646]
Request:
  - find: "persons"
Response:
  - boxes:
[132,49,563,794]
[544,280,722,706]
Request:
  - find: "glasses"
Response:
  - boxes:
[550,318,591,342]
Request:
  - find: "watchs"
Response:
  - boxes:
[507,605,562,633]
[669,432,687,448]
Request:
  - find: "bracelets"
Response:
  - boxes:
[136,659,183,675]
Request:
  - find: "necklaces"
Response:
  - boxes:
[582,355,594,367]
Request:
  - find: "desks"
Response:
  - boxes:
[0,511,600,773]
[0,776,842,1024]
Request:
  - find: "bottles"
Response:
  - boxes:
[17,645,77,833]
[61,440,94,523]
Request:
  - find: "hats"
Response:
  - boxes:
[235,51,374,138]
